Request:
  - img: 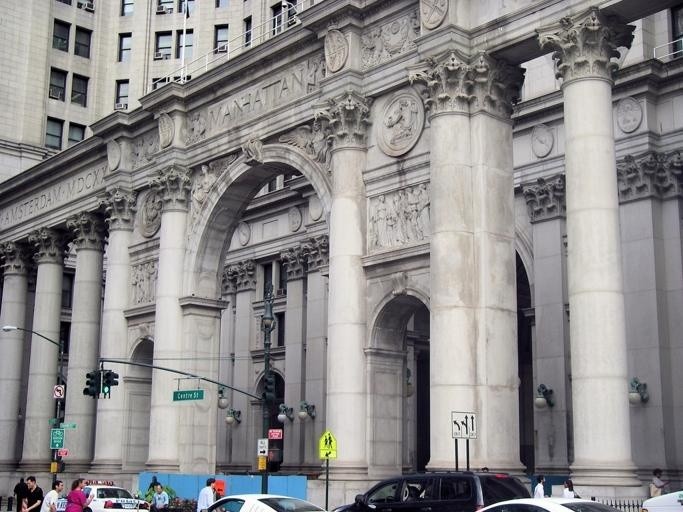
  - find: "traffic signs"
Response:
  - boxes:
[60,422,76,429]
[258,438,269,456]
[172,390,203,402]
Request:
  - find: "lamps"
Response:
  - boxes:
[277,404,293,425]
[533,383,554,409]
[224,409,241,425]
[217,386,228,410]
[297,401,315,422]
[626,375,648,406]
[404,367,413,397]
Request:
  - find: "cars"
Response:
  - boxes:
[477,496,625,512]
[639,490,683,511]
[54,480,150,512]
[200,492,329,512]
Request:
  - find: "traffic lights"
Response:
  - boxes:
[58,462,65,473]
[82,369,100,399]
[261,371,276,404]
[102,370,110,393]
[110,372,118,387]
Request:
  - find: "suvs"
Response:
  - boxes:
[334,470,532,511]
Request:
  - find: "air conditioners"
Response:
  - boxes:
[112,103,127,110]
[154,51,162,59]
[82,2,95,11]
[48,88,61,100]
[155,4,168,14]
[218,44,226,52]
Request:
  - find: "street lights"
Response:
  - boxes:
[258,282,276,489]
[3,325,65,484]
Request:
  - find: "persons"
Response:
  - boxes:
[560,478,576,497]
[23,476,43,512]
[13,478,27,512]
[78,478,87,490]
[150,484,168,512]
[532,474,549,497]
[39,480,63,511]
[649,468,670,497]
[64,477,94,511]
[196,477,222,512]
